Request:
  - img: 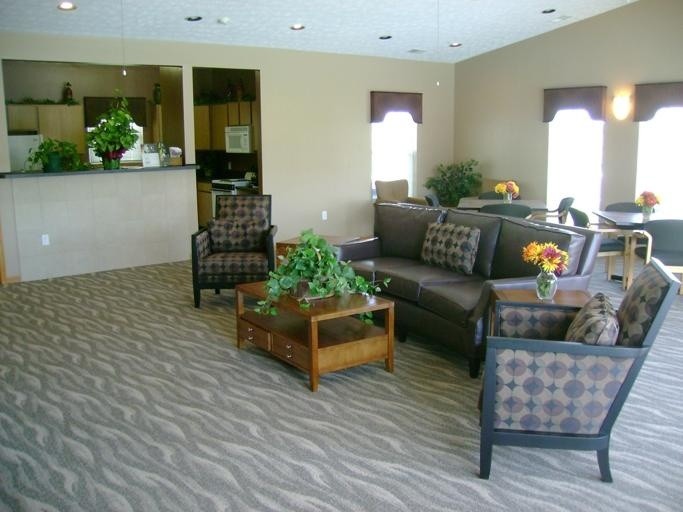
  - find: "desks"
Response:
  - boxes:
[457,197,550,213]
[484,281,593,338]
[272,231,362,271]
[592,208,657,293]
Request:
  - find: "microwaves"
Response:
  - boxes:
[223,125,253,154]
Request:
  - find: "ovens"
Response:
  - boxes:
[210,188,236,219]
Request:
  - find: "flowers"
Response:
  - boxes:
[518,236,572,296]
[494,179,519,200]
[634,191,660,214]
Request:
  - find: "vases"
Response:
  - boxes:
[641,205,652,220]
[501,191,512,203]
[534,270,560,302]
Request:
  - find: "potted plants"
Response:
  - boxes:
[16,132,96,173]
[81,85,141,170]
[249,222,393,326]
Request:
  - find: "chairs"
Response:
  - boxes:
[424,192,444,208]
[564,202,628,292]
[371,176,428,205]
[606,200,643,214]
[530,196,577,229]
[186,193,278,310]
[640,216,682,296]
[462,246,683,487]
[478,190,504,201]
[475,199,535,222]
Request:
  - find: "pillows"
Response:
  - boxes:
[418,219,483,277]
[562,289,622,348]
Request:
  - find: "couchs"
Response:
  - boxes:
[331,193,604,383]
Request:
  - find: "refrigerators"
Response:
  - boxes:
[8,133,44,172]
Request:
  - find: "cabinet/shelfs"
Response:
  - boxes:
[193,104,212,152]
[226,101,252,126]
[6,103,39,133]
[152,105,162,146]
[209,101,228,152]
[195,181,214,228]
[251,97,261,152]
[37,104,87,157]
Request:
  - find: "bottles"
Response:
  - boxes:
[64,83,72,99]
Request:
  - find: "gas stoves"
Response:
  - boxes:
[210,178,251,189]
